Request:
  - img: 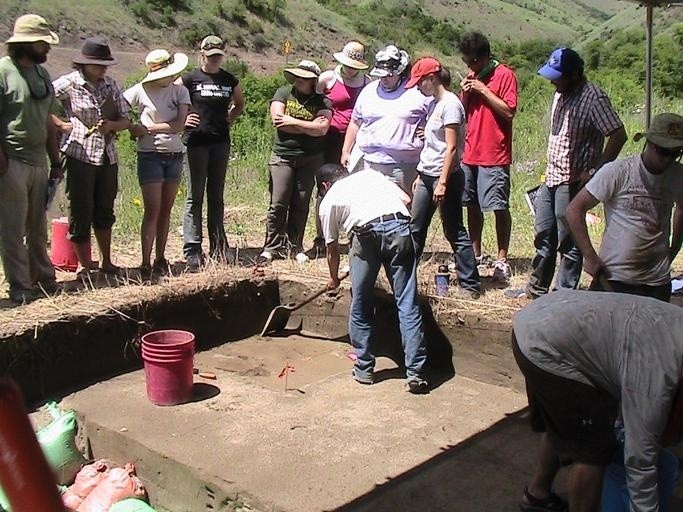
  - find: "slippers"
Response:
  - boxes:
[99,263,127,277]
[77,266,92,283]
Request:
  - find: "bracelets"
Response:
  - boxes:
[146,125,152,136]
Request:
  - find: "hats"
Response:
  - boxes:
[403,56,443,90]
[282,59,321,92]
[141,48,189,83]
[537,47,585,81]
[2,12,59,46]
[72,37,117,67]
[369,44,410,77]
[201,34,226,57]
[332,41,369,70]
[634,112,683,152]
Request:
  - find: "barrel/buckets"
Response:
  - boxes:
[49,218,92,270]
[139,330,196,406]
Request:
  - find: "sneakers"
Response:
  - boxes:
[258,249,274,264]
[8,280,62,303]
[141,258,173,283]
[305,245,326,260]
[209,251,236,264]
[352,363,375,385]
[288,250,310,263]
[504,288,531,303]
[457,284,484,298]
[449,253,489,277]
[188,249,203,271]
[407,376,430,394]
[493,257,512,285]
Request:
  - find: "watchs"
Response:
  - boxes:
[588,166,596,176]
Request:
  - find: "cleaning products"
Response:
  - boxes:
[435,265,450,297]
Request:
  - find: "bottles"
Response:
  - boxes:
[433,263,449,297]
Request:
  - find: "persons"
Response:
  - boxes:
[260,60,334,264]
[504,47,627,300]
[456,32,518,278]
[174,35,245,271]
[122,48,192,280]
[305,42,374,259]
[512,289,682,512]
[0,14,75,303]
[567,112,682,303]
[315,163,434,394]
[49,36,133,282]
[405,57,486,294]
[340,44,434,211]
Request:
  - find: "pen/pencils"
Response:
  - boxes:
[456,71,463,81]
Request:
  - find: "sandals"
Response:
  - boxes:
[520,486,569,512]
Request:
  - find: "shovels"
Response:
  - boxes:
[193,367,216,380]
[260,271,350,337]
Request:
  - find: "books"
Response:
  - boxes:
[524,184,541,216]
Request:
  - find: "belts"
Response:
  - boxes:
[351,212,411,237]
[136,151,184,159]
